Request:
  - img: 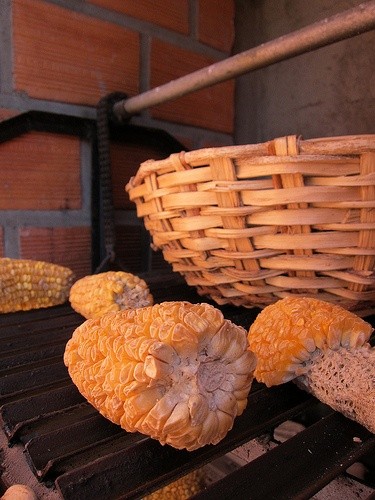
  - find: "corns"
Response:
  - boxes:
[69,271,155,319]
[142,467,208,500]
[63,301,257,451]
[248,294,375,436]
[0,258,77,314]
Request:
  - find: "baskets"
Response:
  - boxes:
[128,132,374,318]
